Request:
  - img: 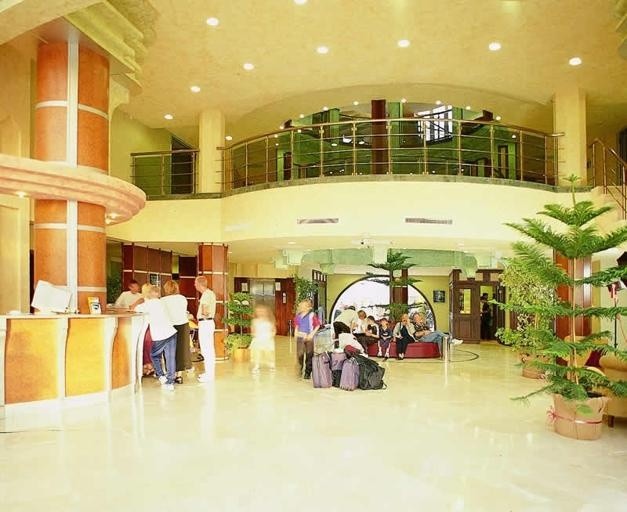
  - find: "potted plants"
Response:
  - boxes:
[364,249,427,331]
[219,292,256,350]
[499,264,554,380]
[487,174,627,441]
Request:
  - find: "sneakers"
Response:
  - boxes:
[304,371,311,379]
[143,368,182,390]
[385,353,404,360]
[198,373,215,382]
[452,339,464,346]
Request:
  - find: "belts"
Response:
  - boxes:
[199,318,213,321]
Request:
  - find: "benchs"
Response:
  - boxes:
[346,341,442,358]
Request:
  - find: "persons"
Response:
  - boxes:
[131,282,178,391]
[114,279,142,306]
[157,280,196,385]
[245,304,277,376]
[332,305,463,359]
[193,276,216,383]
[479,296,491,340]
[292,301,320,380]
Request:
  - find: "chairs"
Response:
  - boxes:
[556,335,608,377]
[599,354,627,427]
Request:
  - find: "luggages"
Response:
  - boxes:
[312,329,385,391]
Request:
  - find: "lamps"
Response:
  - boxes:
[282,292,287,303]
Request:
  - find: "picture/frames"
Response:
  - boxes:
[433,290,445,303]
